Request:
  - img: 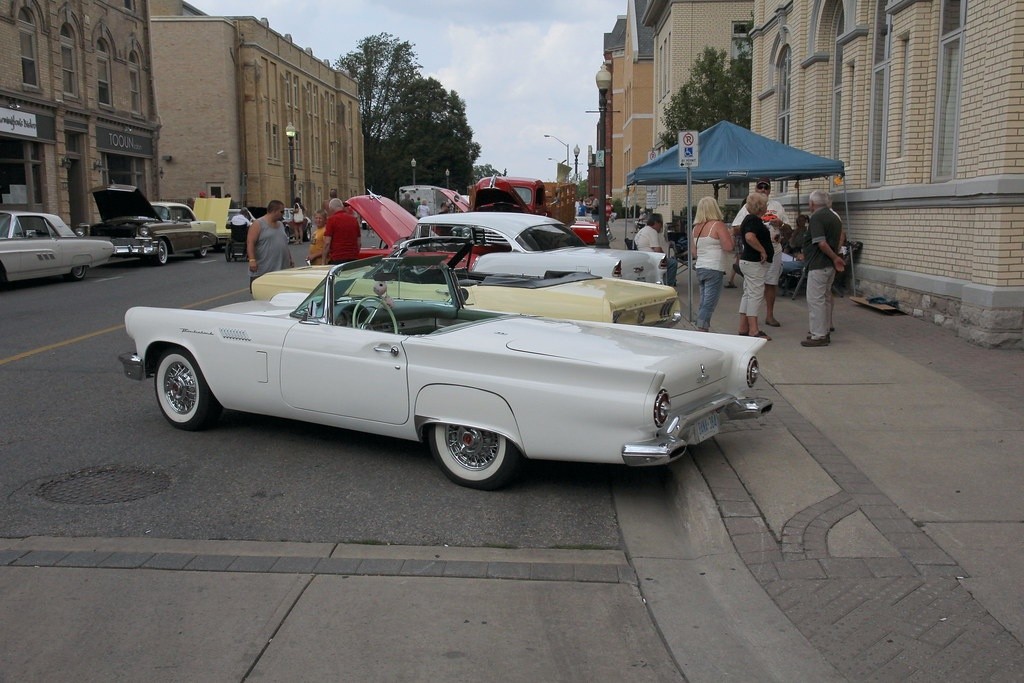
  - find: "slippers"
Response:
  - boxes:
[753,330,772,341]
[738,329,749,336]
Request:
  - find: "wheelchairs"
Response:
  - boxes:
[225,221,250,263]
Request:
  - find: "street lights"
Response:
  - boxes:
[411,159,417,184]
[445,169,452,189]
[544,134,570,167]
[285,121,296,207]
[573,144,580,180]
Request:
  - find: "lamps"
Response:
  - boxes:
[58,156,72,170]
[158,166,164,179]
[93,158,102,174]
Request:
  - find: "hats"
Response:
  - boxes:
[756,178,771,187]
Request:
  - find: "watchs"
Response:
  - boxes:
[778,222,785,230]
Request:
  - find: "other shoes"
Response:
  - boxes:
[830,327,835,332]
[800,337,829,347]
[765,317,780,327]
[293,241,304,245]
[807,334,831,344]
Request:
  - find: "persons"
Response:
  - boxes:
[631,207,647,233]
[231,207,251,227]
[781,215,809,260]
[307,198,362,265]
[689,196,735,332]
[183,191,206,220]
[291,197,308,244]
[730,177,791,341]
[214,193,237,209]
[578,194,617,242]
[246,199,295,301]
[800,190,847,347]
[632,212,678,288]
[400,192,450,219]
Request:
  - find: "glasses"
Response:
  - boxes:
[755,184,770,189]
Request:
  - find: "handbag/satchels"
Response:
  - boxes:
[293,202,304,223]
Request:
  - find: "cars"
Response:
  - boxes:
[346,190,670,286]
[119,249,773,492]
[210,208,258,252]
[436,175,614,245]
[251,236,682,329]
[85,183,218,266]
[282,208,313,245]
[0,210,115,290]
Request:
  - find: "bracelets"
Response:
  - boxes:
[247,257,257,264]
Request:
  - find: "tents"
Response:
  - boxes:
[625,121,857,322]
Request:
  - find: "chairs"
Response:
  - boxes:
[625,209,863,300]
